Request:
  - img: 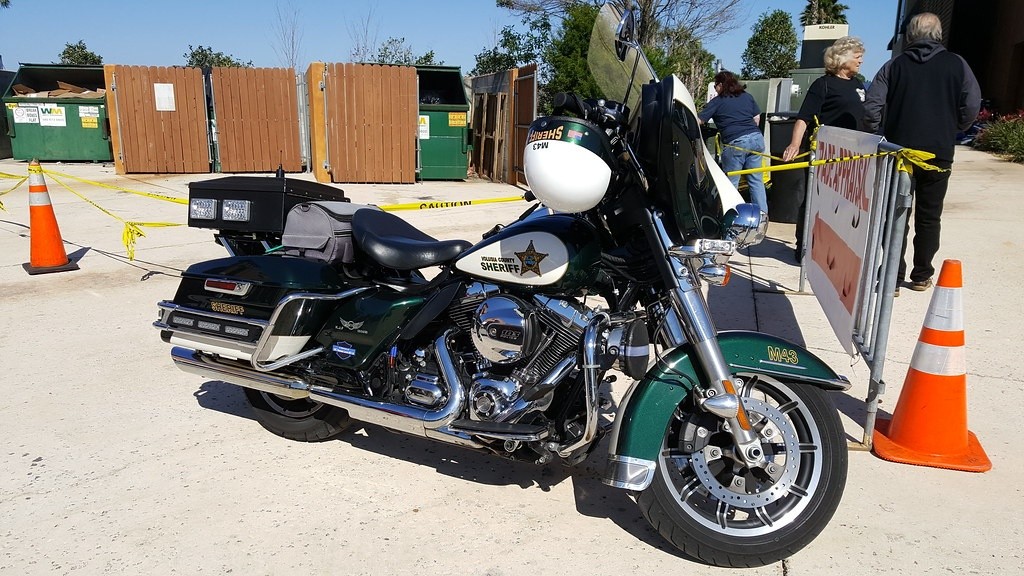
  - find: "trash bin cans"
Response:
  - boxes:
[416,66,470,179]
[700,121,717,159]
[766,111,804,223]
[1,62,113,163]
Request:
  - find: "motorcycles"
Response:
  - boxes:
[153,8,851,568]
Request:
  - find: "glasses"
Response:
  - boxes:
[714,82,721,89]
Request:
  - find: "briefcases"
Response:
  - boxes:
[188,176,351,233]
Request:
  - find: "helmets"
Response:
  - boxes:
[523,117,619,214]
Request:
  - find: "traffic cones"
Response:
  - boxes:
[22,158,80,275]
[872,260,992,473]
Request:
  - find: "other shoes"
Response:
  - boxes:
[912,278,935,291]
[794,253,802,267]
[876,282,900,297]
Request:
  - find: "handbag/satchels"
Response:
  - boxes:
[282,201,384,266]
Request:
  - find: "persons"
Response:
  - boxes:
[866,12,982,297]
[697,71,768,241]
[783,36,868,263]
